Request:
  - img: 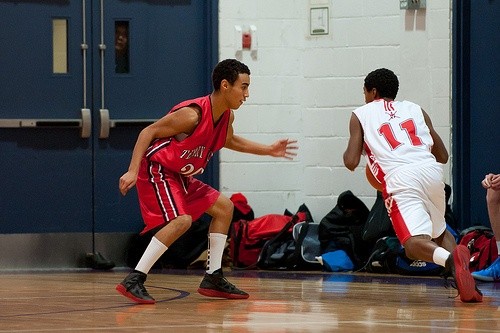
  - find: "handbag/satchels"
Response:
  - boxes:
[123,190,499,277]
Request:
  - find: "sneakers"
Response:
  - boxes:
[197,268,249,299]
[115,270,156,304]
[439,244,482,302]
[471,256,500,282]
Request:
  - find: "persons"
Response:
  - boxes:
[343,68,482,302]
[116,59,299,304]
[471,172,500,282]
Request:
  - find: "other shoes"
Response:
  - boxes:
[84,251,115,270]
